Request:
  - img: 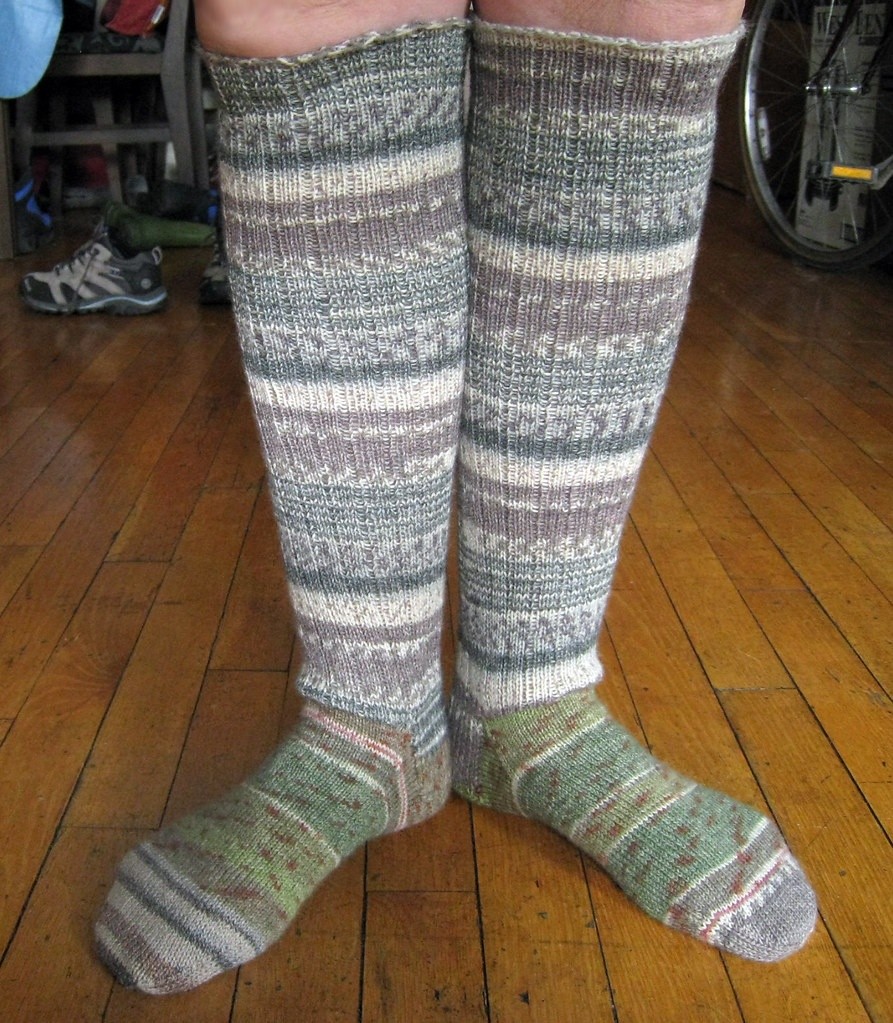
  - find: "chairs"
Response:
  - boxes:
[0,0,208,258]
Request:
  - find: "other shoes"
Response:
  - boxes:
[64,169,109,208]
[14,167,53,253]
[136,179,202,221]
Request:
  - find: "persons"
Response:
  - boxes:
[82,0,819,993]
[0,0,64,252]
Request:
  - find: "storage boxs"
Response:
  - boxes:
[795,5,886,251]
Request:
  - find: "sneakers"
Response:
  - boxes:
[199,221,234,306]
[20,223,168,316]
